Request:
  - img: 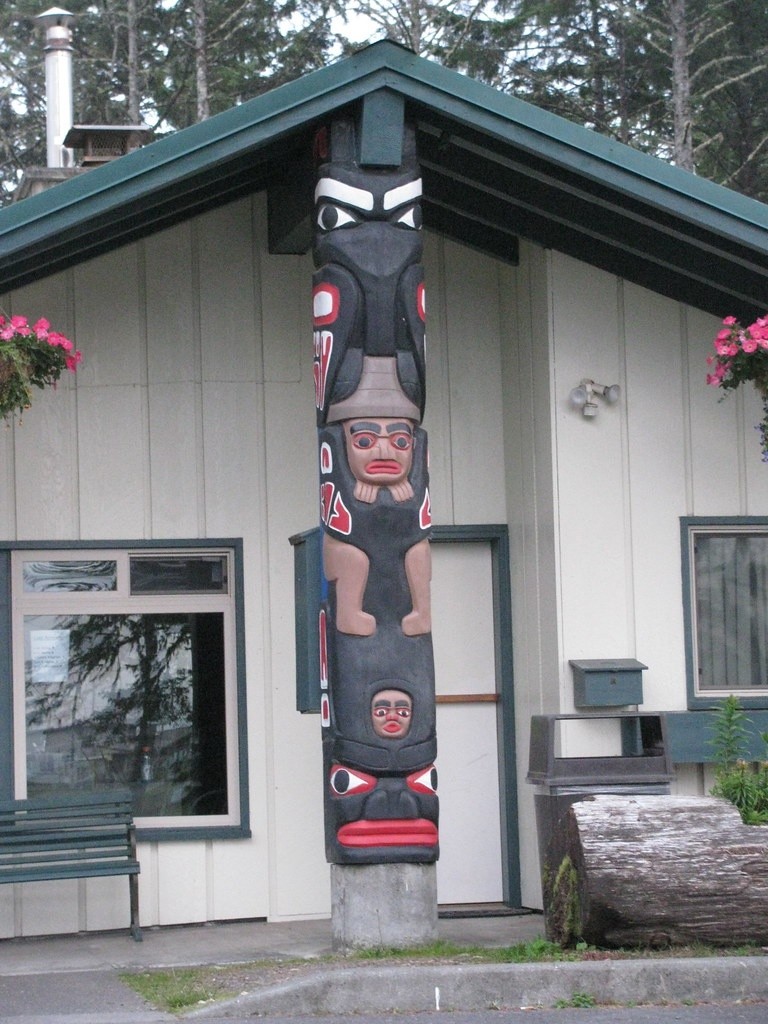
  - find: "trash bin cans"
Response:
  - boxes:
[526,710,677,939]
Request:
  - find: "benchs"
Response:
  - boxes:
[2,784,148,944]
